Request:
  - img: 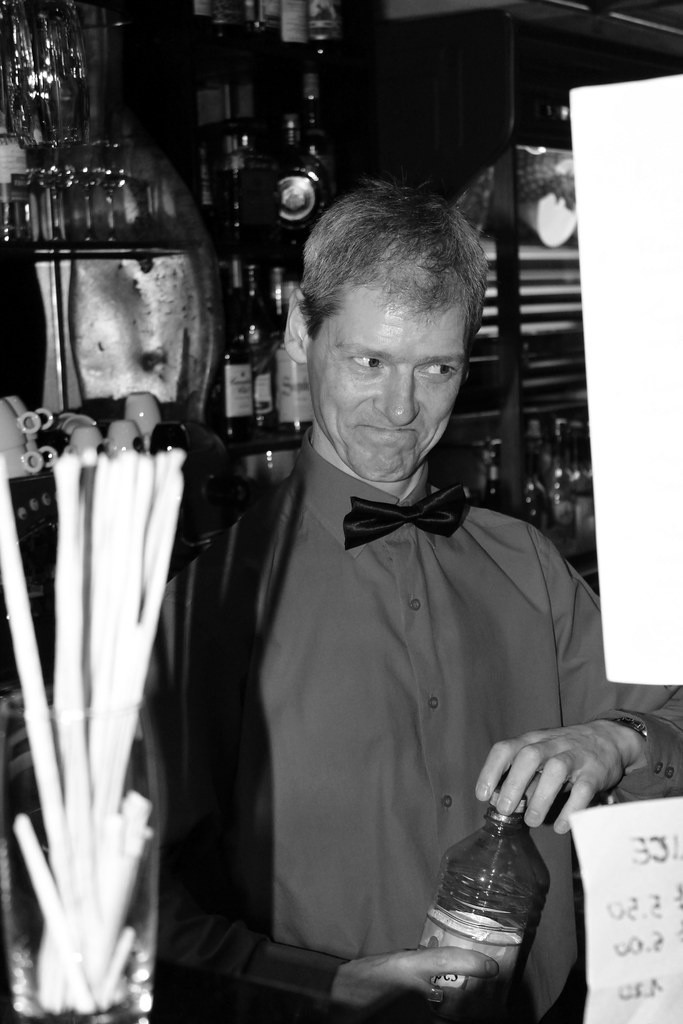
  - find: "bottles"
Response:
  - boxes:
[182,1,343,434]
[415,788,551,1024]
[471,417,594,552]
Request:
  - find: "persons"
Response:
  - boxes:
[167,199,683,1010]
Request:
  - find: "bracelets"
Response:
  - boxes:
[617,717,647,736]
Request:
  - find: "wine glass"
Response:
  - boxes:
[27,140,132,241]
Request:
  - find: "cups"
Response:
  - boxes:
[0,391,168,481]
[0,806,166,1024]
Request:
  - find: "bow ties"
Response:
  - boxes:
[343,484,466,551]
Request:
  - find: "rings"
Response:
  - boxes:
[428,986,443,1009]
